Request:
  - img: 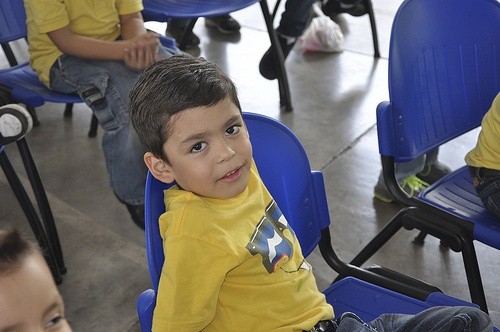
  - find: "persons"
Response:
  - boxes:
[260,0,368,80]
[374,145,452,207]
[463,86,500,219]
[129,53,496,332]
[23,0,182,229]
[165,14,242,51]
[0,225,73,332]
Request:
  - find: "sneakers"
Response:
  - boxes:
[418,162,453,185]
[373,173,430,205]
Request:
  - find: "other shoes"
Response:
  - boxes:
[166,24,200,49]
[205,13,241,34]
[259,34,298,79]
[114,191,145,229]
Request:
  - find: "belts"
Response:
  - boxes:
[301,319,336,332]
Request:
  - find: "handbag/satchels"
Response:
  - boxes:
[301,5,344,54]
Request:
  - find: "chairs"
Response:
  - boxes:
[330,0,500,315]
[0,0,381,293]
[136,112,500,332]
